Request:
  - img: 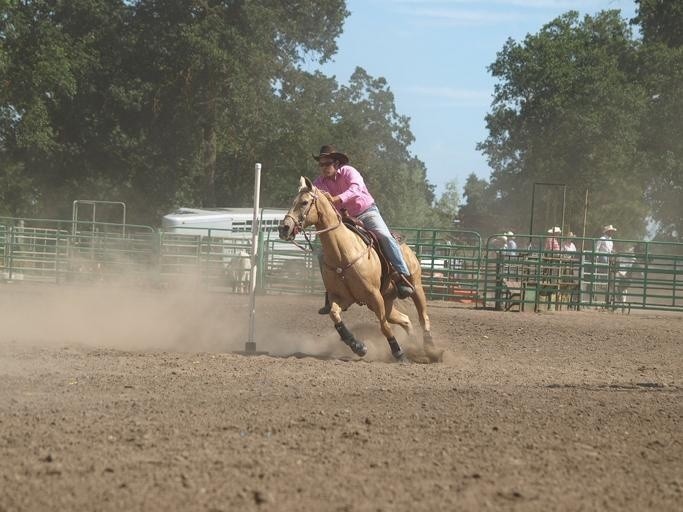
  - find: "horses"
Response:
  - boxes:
[229,251,252,295]
[579,242,655,306]
[277,174,442,365]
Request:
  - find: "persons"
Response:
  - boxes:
[504,232,518,255]
[544,224,561,251]
[312,145,416,315]
[560,230,579,259]
[594,224,617,279]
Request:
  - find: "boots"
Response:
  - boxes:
[319,292,331,314]
[399,277,413,298]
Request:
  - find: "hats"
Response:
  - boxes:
[603,225,616,234]
[492,231,515,243]
[547,227,576,237]
[312,145,349,165]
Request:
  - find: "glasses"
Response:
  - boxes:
[319,160,336,167]
